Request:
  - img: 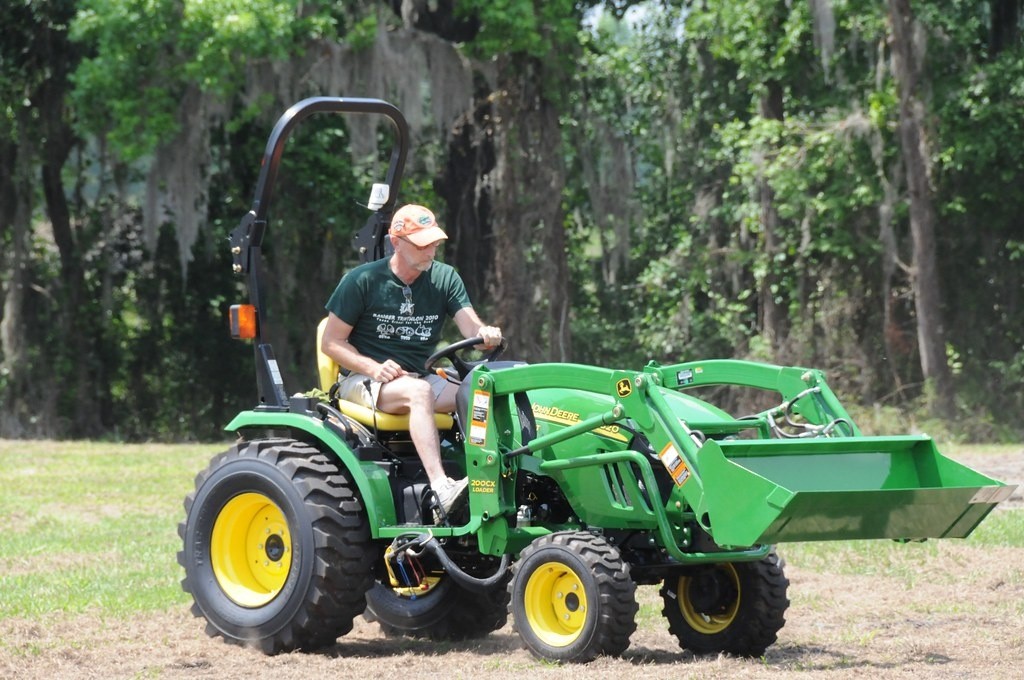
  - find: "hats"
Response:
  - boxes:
[389,204,448,247]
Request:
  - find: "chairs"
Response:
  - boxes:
[315,314,455,434]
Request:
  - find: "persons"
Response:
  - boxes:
[320,204,501,526]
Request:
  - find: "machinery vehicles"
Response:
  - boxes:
[175,93,1018,665]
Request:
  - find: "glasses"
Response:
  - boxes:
[394,235,440,250]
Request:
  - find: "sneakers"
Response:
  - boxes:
[431,475,468,526]
